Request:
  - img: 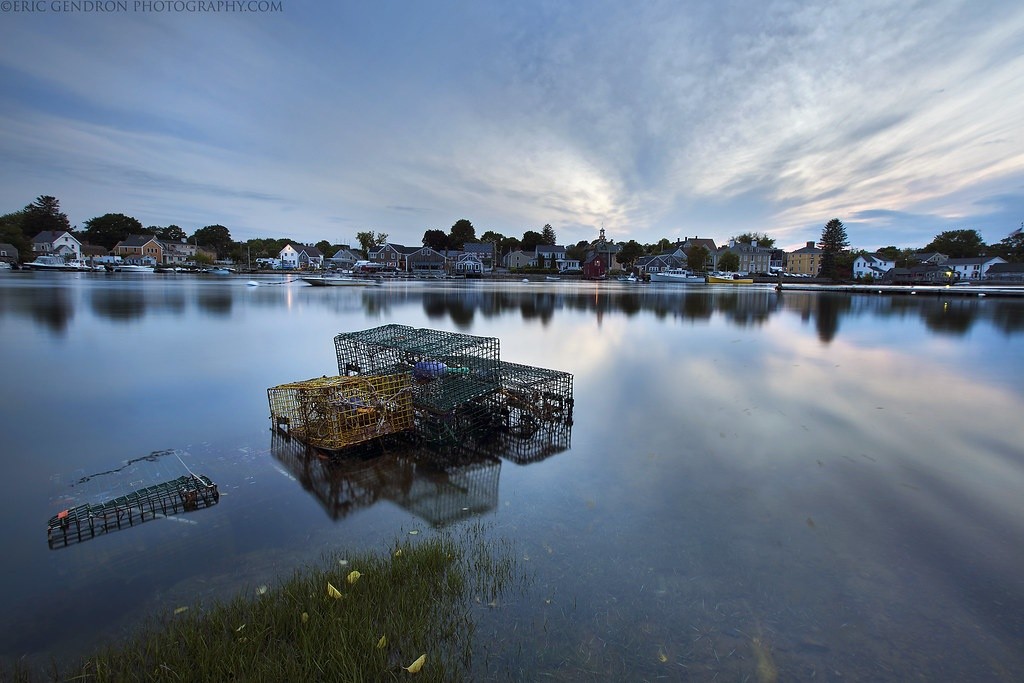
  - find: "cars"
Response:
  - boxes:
[760,271,811,278]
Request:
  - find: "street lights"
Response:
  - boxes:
[978,251,986,281]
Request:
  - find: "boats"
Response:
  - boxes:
[0,269,756,288]
